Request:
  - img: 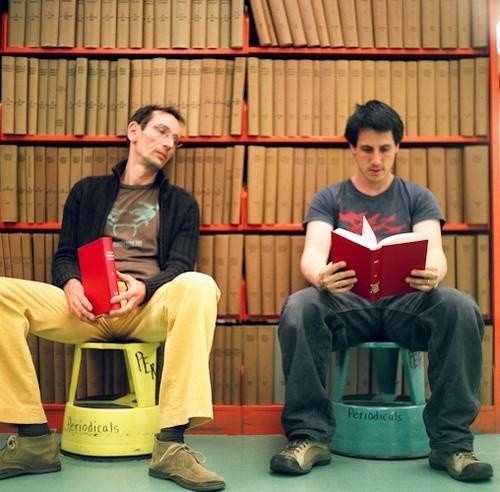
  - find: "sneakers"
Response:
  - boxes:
[270,438,331,475]
[428,449,493,482]
[0,428,62,480]
[148,432,225,491]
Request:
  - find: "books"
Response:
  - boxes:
[3,1,492,408]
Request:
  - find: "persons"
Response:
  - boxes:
[1,104,226,492]
[272,100,495,484]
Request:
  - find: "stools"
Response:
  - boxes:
[60,342,165,458]
[328,341,435,460]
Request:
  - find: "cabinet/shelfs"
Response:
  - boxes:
[244,0,498,435]
[0,0,247,437]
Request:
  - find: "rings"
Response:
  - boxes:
[427,279,430,284]
